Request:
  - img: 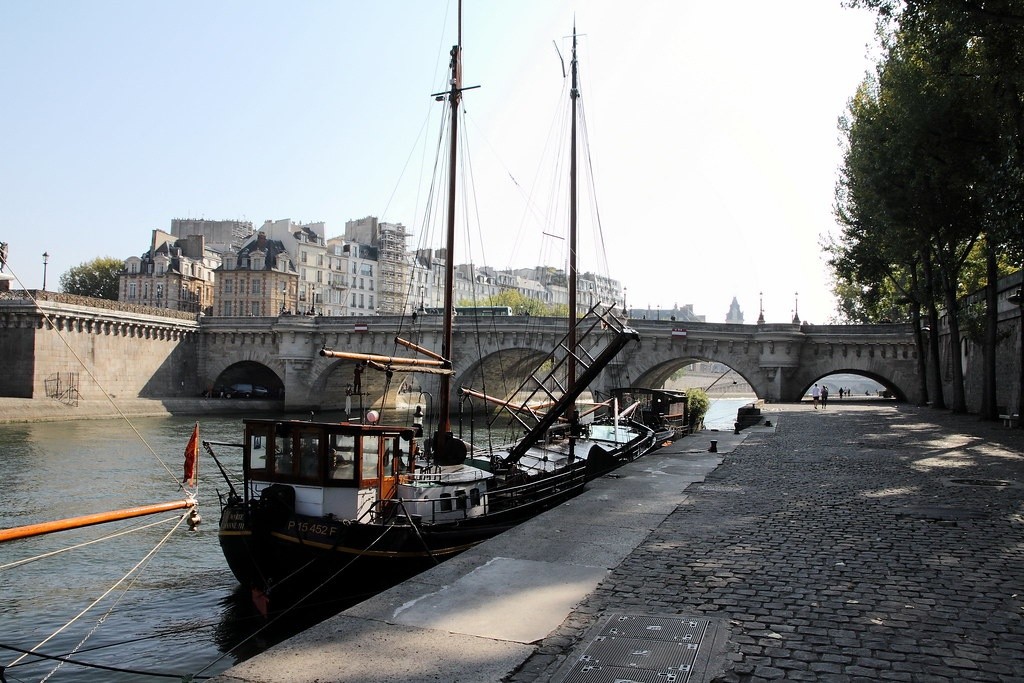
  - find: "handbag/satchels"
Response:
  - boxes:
[844,390,847,393]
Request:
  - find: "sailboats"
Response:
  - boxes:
[218,1,655,587]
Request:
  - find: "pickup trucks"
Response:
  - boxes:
[879,389,895,398]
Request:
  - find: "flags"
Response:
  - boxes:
[180,424,198,486]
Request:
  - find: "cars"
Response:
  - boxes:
[212,384,268,399]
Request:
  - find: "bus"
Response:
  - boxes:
[416,307,512,316]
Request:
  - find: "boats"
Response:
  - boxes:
[609,387,705,450]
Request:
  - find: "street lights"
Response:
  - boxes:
[157,285,161,308]
[311,286,316,314]
[588,283,594,313]
[792,293,801,324]
[757,293,766,324]
[42,252,49,291]
[657,305,660,320]
[622,287,628,314]
[417,285,427,315]
[282,288,287,312]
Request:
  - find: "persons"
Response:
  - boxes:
[865,390,869,396]
[276,448,337,478]
[839,388,844,398]
[821,386,828,409]
[303,311,306,315]
[525,310,530,315]
[813,384,821,409]
[848,388,851,396]
[287,309,291,314]
[671,315,676,320]
[307,311,310,314]
[844,386,847,395]
[517,311,522,315]
[296,310,301,314]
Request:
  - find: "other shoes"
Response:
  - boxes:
[815,407,817,409]
[824,407,826,409]
[822,407,823,409]
